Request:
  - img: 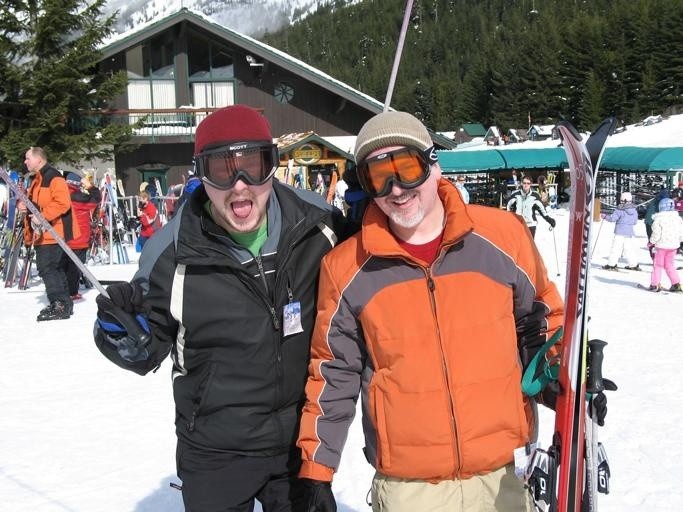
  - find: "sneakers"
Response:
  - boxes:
[37,301,73,319]
[650,283,682,292]
[602,264,639,270]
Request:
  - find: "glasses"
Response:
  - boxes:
[356,147,430,197]
[197,145,280,190]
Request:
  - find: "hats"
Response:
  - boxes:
[194,105,272,158]
[355,111,438,164]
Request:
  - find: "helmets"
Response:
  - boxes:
[658,197,674,211]
[620,192,632,204]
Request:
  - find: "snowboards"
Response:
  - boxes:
[283,165,344,218]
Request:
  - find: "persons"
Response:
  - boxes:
[506,174,556,240]
[15,145,74,321]
[63,172,101,301]
[94,103,356,511]
[454,174,469,204]
[286,107,617,512]
[134,191,163,252]
[593,178,683,294]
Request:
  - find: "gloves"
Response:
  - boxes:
[305,482,336,512]
[594,379,617,426]
[550,219,555,227]
[647,241,654,248]
[96,283,151,337]
